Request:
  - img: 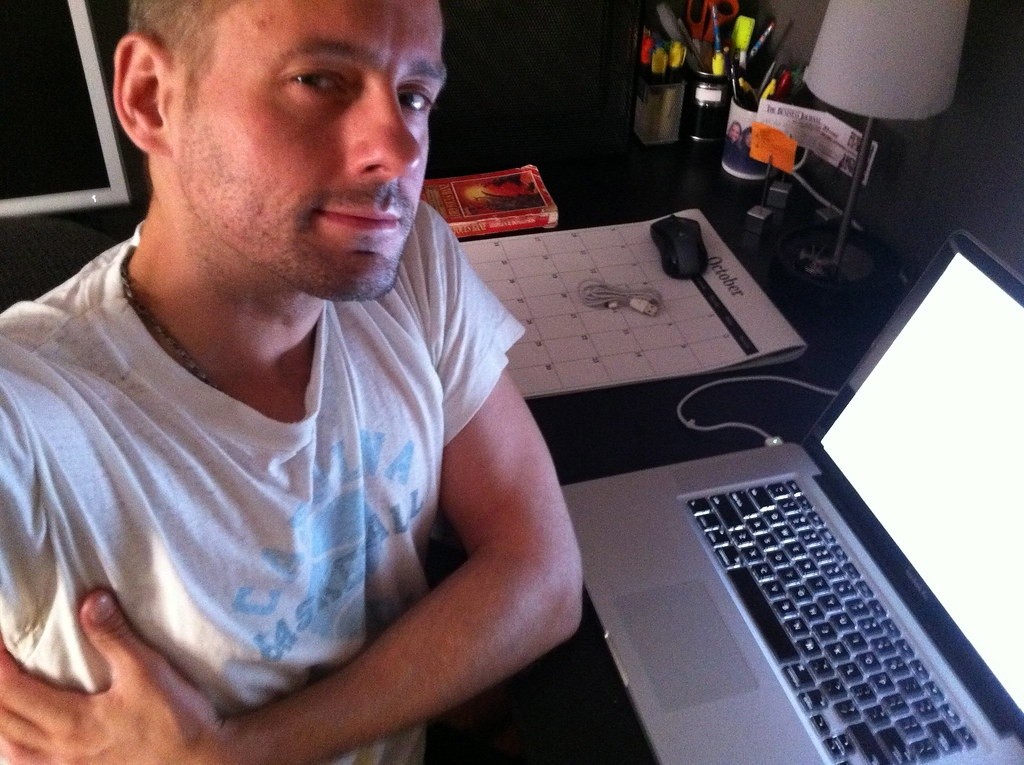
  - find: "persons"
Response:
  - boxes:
[0,0,585,764]
[723,121,766,176]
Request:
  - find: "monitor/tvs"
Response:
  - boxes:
[0,0,130,217]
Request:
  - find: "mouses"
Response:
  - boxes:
[651,216,708,279]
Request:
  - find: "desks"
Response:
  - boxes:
[0,115,1024,765]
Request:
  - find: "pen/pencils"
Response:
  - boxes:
[701,9,775,72]
[730,61,808,111]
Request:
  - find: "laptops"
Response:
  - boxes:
[559,228,1024,765]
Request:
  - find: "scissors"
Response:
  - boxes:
[687,0,739,58]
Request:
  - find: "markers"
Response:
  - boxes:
[640,31,687,84]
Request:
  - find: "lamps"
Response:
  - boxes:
[802,0,971,286]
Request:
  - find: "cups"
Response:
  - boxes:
[634,74,686,146]
[687,70,731,142]
[722,96,810,181]
